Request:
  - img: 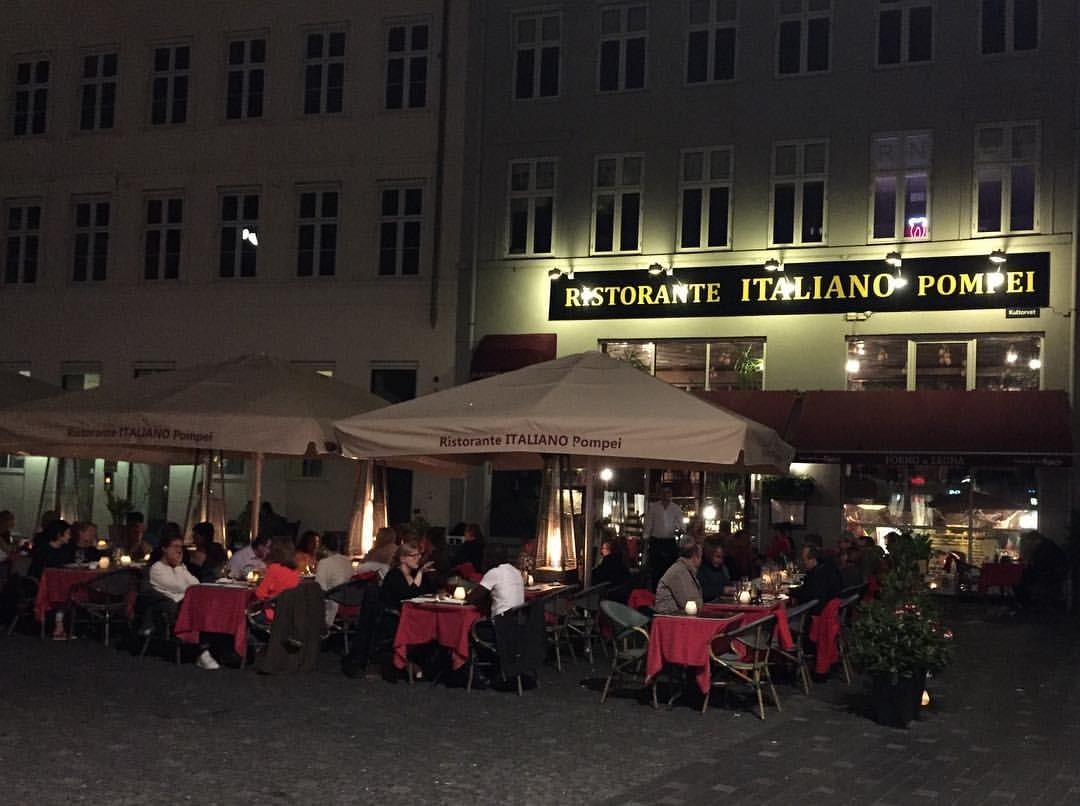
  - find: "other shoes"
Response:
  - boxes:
[53,632,78,640]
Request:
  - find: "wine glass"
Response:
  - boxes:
[737,558,800,604]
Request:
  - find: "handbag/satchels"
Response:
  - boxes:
[474,621,495,662]
[637,606,657,639]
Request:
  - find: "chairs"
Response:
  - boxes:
[8,535,866,721]
[949,551,979,599]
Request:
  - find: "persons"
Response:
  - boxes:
[190,481,209,524]
[1013,531,1068,609]
[563,515,918,700]
[0,501,525,690]
[642,487,687,595]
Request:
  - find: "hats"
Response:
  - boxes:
[196,522,214,540]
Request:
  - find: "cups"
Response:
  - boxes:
[88,562,97,569]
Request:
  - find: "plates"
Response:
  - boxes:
[304,575,316,578]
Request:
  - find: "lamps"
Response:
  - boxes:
[322,441,342,453]
[1029,351,1042,370]
[163,482,168,489]
[765,259,785,273]
[989,250,1008,271]
[102,471,111,484]
[1005,344,1019,364]
[887,253,904,275]
[549,268,576,281]
[648,264,674,278]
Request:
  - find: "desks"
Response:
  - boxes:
[393,579,570,690]
[175,558,377,669]
[644,610,746,715]
[703,586,801,655]
[34,560,144,640]
[979,561,1023,603]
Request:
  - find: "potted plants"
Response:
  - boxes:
[847,589,945,723]
[734,344,757,389]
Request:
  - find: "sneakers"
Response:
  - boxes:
[196,650,221,670]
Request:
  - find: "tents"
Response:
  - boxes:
[0,354,391,540]
[331,351,796,659]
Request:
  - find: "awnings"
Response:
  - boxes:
[793,389,1074,467]
[470,334,556,372]
[686,391,796,449]
